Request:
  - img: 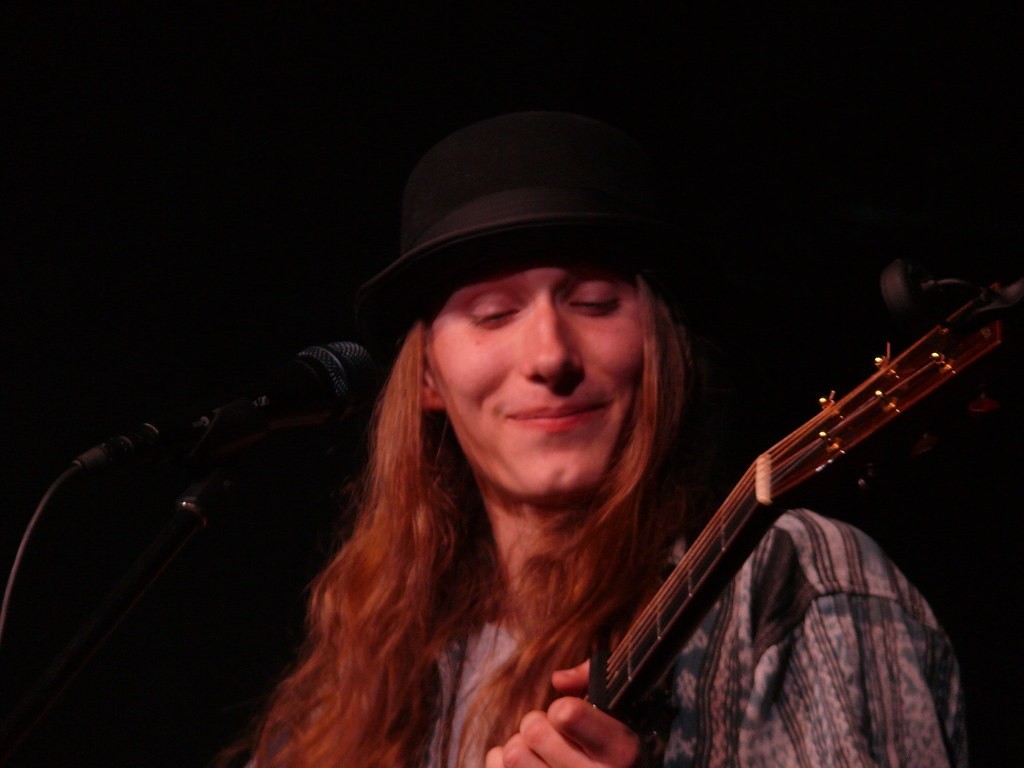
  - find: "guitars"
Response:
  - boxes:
[548,256,1024,716]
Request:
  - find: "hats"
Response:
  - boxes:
[354,105,726,361]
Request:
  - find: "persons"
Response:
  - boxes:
[214,111,968,767]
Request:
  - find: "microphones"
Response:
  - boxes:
[71,342,372,474]
